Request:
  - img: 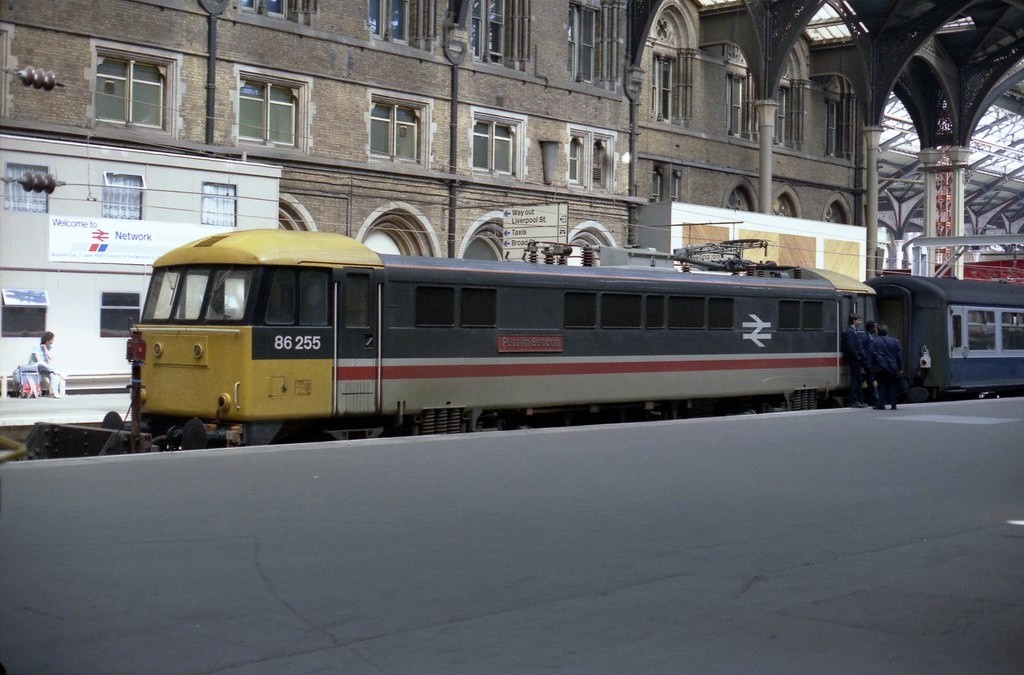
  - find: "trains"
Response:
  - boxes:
[132,229,1024,463]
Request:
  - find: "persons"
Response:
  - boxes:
[869,325,903,410]
[841,314,878,408]
[29,332,70,399]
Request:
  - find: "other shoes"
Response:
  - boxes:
[872,405,885,409]
[851,401,867,407]
[891,405,896,410]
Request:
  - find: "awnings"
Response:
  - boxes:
[2,287,48,307]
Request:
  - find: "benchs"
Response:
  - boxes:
[0,372,66,399]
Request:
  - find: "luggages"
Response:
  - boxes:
[14,364,42,398]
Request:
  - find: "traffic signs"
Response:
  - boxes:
[503,205,570,226]
[502,249,569,264]
[503,236,569,248]
[503,228,569,237]
